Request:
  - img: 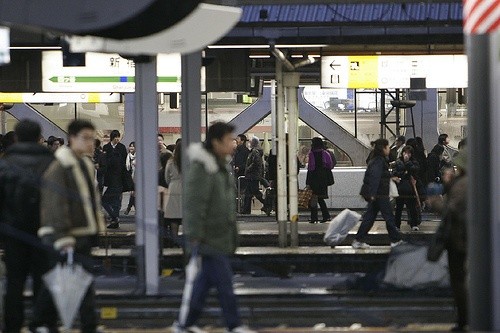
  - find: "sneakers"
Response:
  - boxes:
[351,239,370,249]
[390,240,408,248]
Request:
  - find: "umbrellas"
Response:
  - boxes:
[43,245,94,331]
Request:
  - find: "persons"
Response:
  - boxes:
[0,118,471,333]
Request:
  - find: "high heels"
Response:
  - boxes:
[320,215,330,223]
[310,218,318,223]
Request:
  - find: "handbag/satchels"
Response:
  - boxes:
[298,187,312,209]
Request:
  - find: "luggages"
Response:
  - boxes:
[323,208,361,249]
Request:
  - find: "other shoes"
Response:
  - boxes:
[412,226,419,231]
[225,326,256,333]
[172,322,208,333]
[106,217,120,228]
